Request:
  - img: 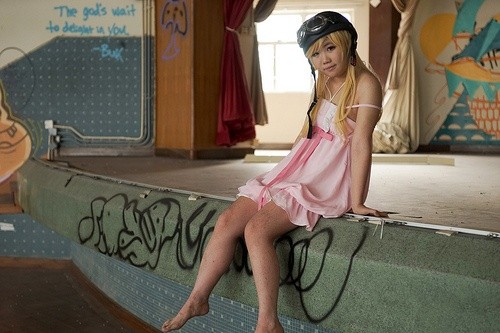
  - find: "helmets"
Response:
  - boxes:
[298,11,358,54]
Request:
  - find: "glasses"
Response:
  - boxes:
[297,15,334,45]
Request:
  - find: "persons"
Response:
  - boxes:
[160,11,389,333]
